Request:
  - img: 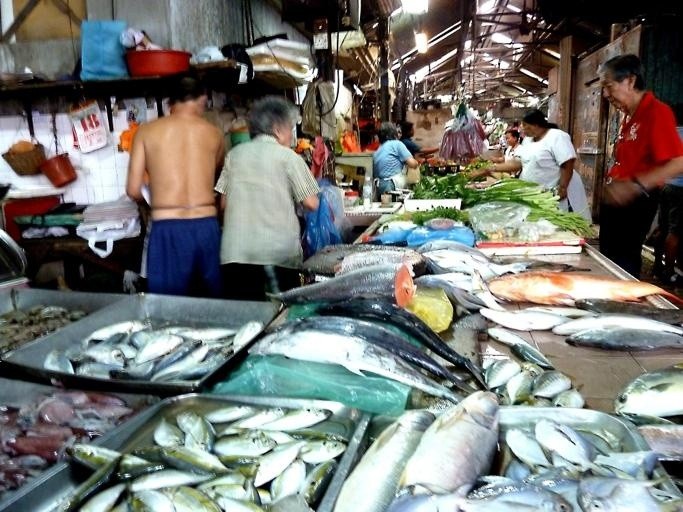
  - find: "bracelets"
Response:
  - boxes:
[632,178,647,194]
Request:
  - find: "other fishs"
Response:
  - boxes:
[0,239,682,512]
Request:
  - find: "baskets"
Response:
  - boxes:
[2,138,45,175]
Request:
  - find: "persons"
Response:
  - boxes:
[652,126,683,283]
[126,76,226,296]
[373,108,593,226]
[214,95,321,301]
[595,53,683,280]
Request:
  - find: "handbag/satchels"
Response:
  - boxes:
[76,218,141,260]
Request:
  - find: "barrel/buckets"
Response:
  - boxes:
[41,136,78,188]
[5,198,60,242]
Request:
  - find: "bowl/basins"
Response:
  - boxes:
[120,48,193,76]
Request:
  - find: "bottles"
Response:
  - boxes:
[361,175,373,211]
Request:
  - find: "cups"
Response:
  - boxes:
[37,153,77,187]
[378,194,392,209]
[343,192,359,212]
